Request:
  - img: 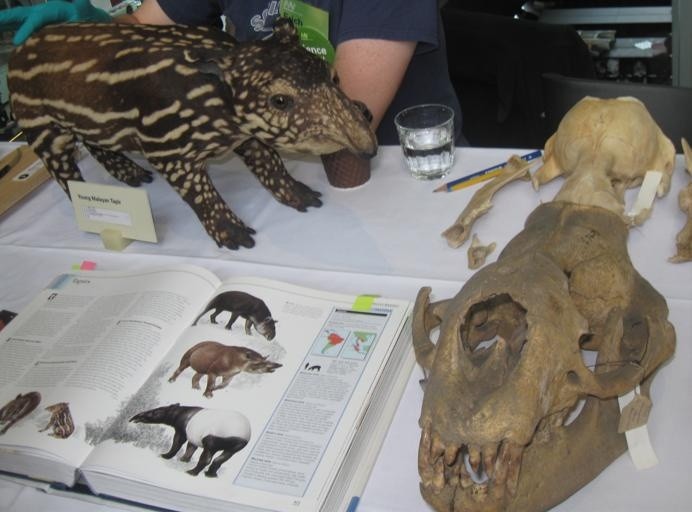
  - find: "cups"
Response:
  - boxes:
[320,100,374,189]
[395,103,455,182]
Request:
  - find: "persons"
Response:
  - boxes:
[0,1,471,147]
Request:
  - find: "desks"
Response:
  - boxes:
[0,145,692,512]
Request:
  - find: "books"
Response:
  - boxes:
[0,270,430,512]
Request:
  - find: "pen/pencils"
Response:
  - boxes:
[433,148,542,194]
